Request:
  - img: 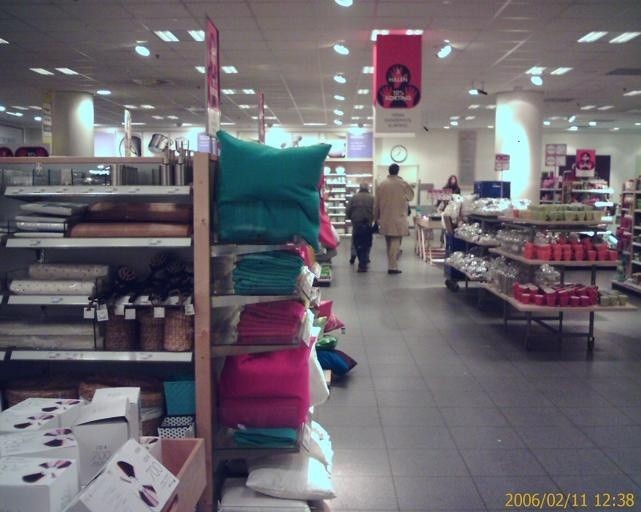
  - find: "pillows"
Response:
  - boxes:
[214,129,332,245]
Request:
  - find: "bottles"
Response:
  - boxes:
[493,226,523,296]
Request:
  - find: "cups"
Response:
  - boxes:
[512,281,629,308]
[523,235,619,262]
[516,203,603,221]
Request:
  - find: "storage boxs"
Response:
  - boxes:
[2,386,210,512]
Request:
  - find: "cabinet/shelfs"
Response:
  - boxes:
[322,157,377,236]
[2,157,309,510]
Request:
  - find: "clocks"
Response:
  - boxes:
[392,145,407,162]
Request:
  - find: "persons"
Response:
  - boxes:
[433,175,461,247]
[345,180,379,272]
[372,163,414,274]
[349,234,358,264]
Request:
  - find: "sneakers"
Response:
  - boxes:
[350,255,370,272]
[388,270,401,273]
